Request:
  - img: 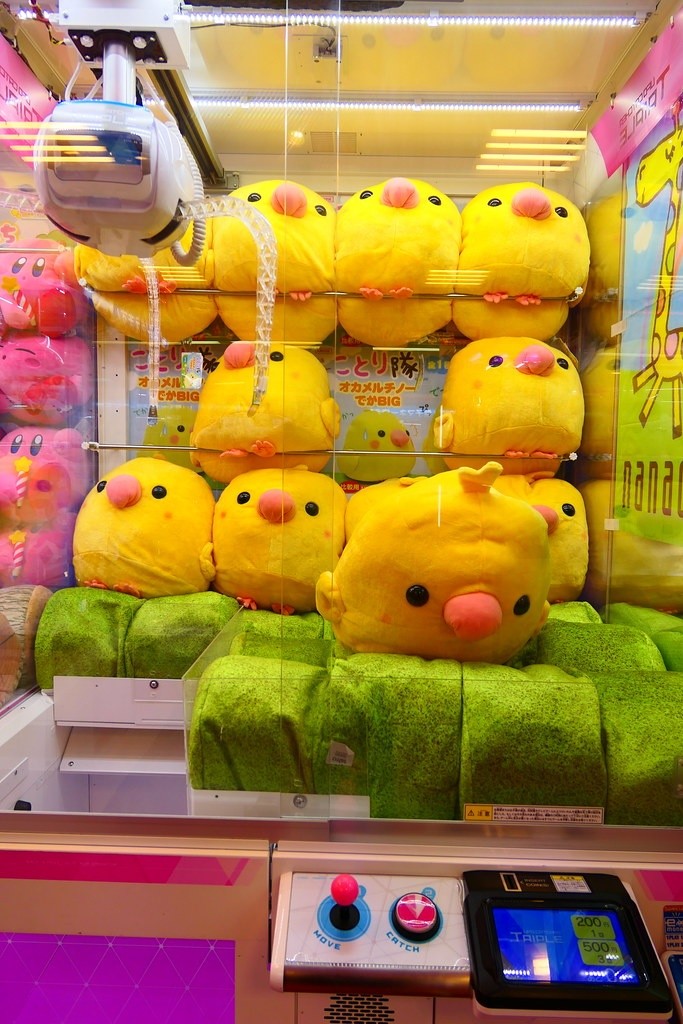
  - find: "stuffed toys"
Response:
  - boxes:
[76,179,593,665]
[0,239,99,589]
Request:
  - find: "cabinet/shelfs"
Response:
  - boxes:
[82,284,587,464]
